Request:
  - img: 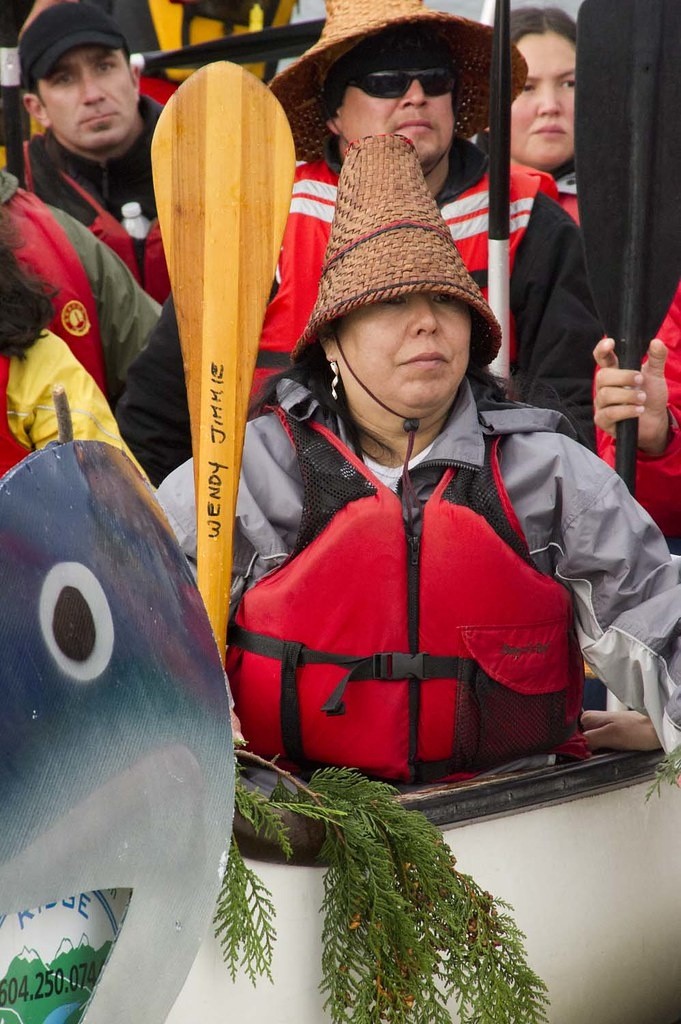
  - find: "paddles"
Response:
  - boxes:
[148,54,299,657]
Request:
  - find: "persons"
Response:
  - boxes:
[154,134,681,796]
[0,0,681,557]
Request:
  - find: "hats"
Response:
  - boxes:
[288,131,503,373]
[18,2,130,93]
[264,0,528,162]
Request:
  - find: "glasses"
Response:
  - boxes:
[347,67,459,99]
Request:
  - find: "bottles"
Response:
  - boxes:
[120,201,152,240]
